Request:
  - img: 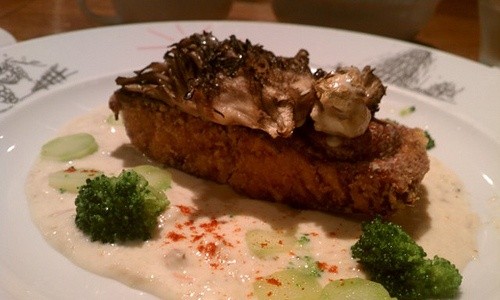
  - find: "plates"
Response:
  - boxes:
[0,17,497,300]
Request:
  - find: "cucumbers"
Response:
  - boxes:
[247,227,390,300]
[42,132,172,194]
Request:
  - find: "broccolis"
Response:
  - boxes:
[74,167,171,244]
[350,213,464,300]
